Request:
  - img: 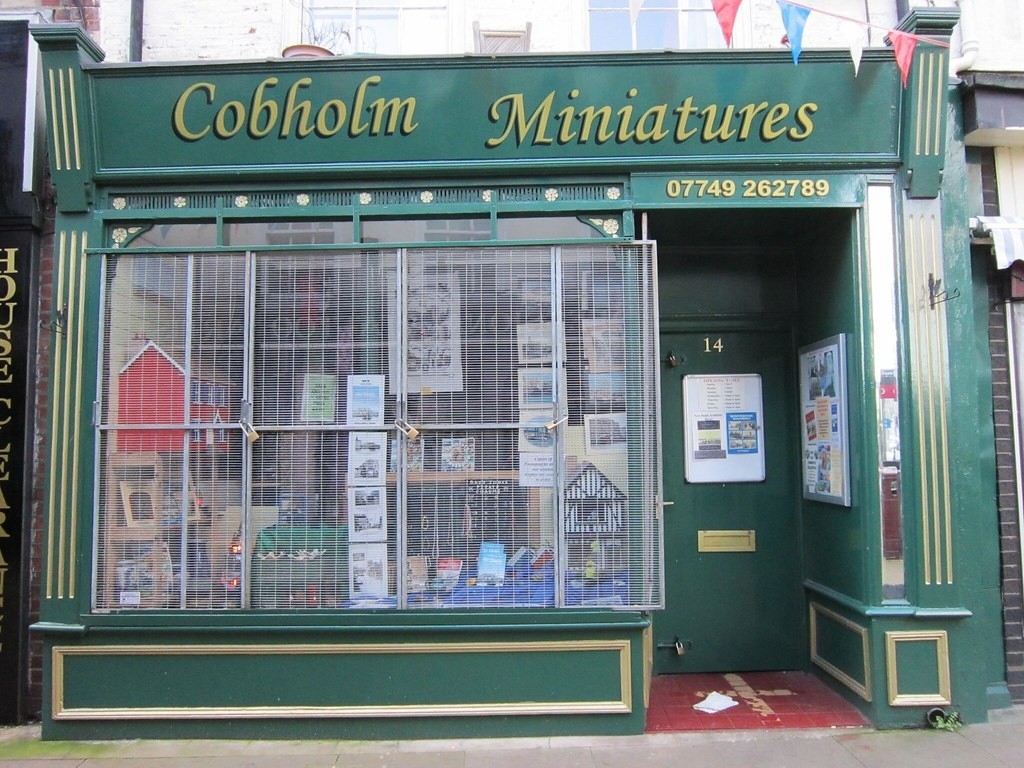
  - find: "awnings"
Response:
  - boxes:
[976,215,1024,270]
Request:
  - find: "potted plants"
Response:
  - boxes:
[282,0,347,57]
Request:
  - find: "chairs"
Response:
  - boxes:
[105,452,213,608]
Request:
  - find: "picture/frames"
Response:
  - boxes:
[380,267,473,400]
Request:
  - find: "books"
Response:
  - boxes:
[436,559,463,590]
[406,556,429,593]
[477,543,506,587]
